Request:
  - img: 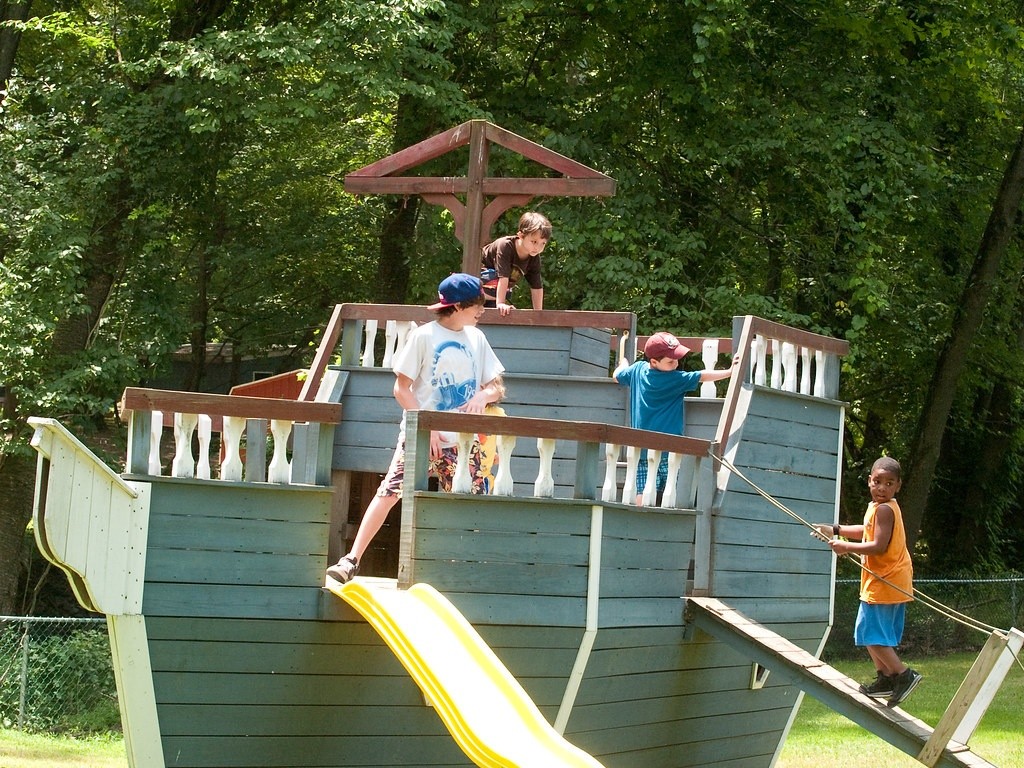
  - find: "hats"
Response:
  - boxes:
[643,330,691,360]
[426,272,484,310]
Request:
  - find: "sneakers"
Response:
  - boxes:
[858,669,895,698]
[327,553,361,584]
[887,667,922,709]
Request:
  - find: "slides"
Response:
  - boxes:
[327,581,605,768]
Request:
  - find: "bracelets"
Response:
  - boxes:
[833,523,840,539]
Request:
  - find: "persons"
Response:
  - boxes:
[451,210,552,314]
[810,456,922,709]
[611,331,740,505]
[326,272,504,583]
